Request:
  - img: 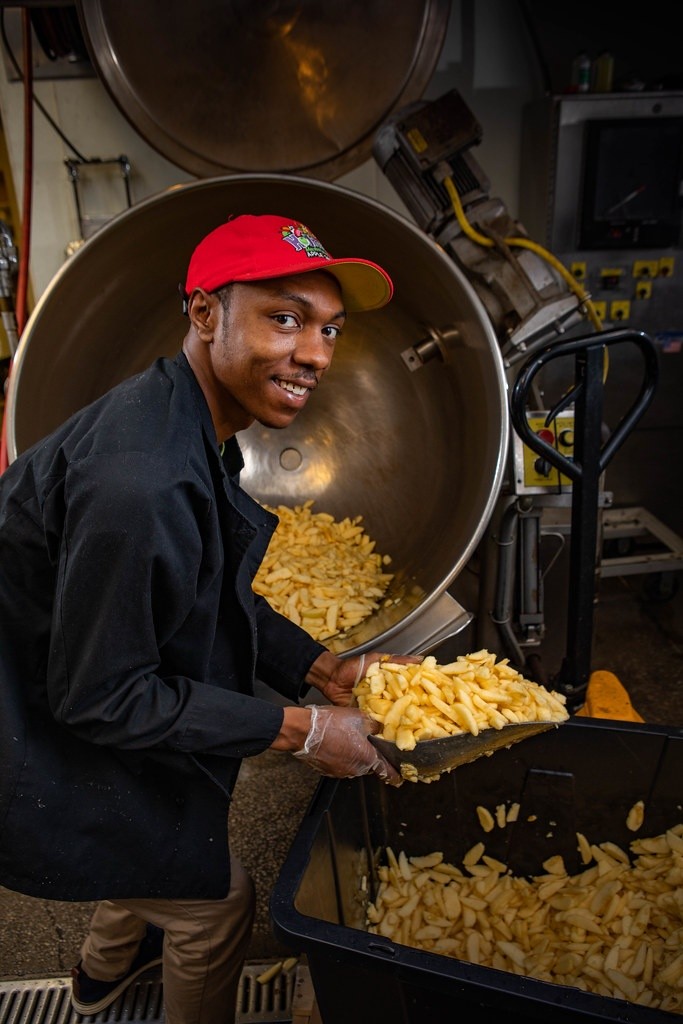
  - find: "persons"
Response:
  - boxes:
[0,214,426,1024]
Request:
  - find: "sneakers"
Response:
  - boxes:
[71,922,165,1016]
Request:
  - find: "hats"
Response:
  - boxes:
[182,214,394,314]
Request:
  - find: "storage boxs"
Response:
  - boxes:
[269,716,683,1024]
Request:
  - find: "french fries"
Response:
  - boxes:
[250,499,394,640]
[352,648,571,751]
[364,801,683,1016]
